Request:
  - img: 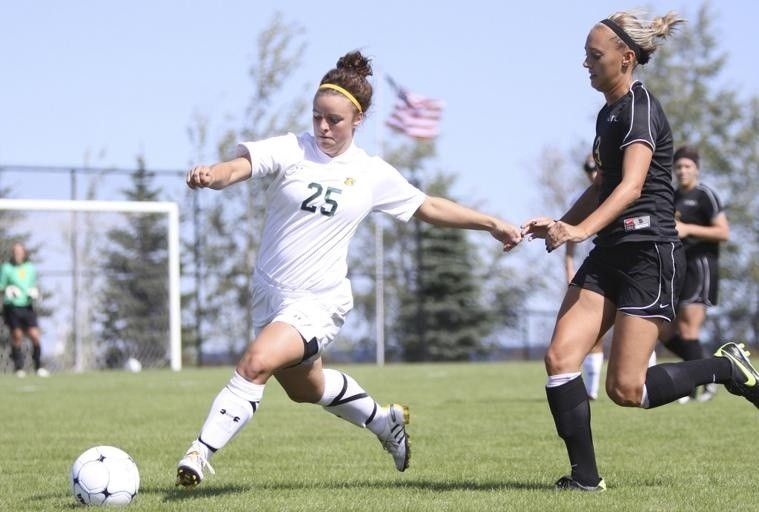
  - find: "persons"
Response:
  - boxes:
[518,8,759,493]
[1,242,49,377]
[663,144,730,406]
[563,151,657,411]
[172,52,526,486]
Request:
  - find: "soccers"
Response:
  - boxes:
[72,446,140,506]
[124,358,144,372]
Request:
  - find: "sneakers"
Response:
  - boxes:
[698,382,716,402]
[554,475,607,494]
[175,440,215,487]
[377,404,411,472]
[712,342,759,410]
